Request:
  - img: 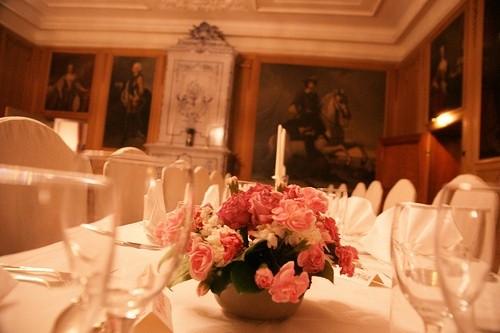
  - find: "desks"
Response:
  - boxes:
[0,223,500,333]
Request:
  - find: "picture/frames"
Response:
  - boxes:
[36,50,100,119]
[240,56,392,197]
[424,2,469,127]
[91,51,165,151]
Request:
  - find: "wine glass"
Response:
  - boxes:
[63,148,197,332]
[393,201,491,333]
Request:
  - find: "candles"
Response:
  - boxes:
[280,128,287,176]
[275,124,281,176]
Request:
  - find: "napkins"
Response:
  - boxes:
[357,203,463,286]
[201,183,220,212]
[143,176,167,234]
[328,193,377,238]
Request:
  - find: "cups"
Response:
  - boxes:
[0,163,120,333]
[435,182,500,333]
[186,128,197,146]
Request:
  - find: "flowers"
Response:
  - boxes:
[154,181,361,303]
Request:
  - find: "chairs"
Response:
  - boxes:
[0,117,500,272]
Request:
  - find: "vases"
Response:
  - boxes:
[213,282,303,322]
